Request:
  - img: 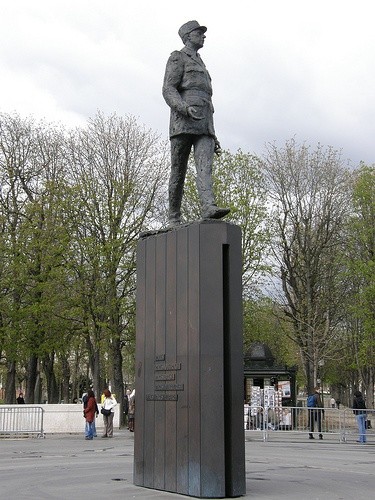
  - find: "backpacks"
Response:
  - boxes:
[307,394,317,407]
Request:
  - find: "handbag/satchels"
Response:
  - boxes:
[129,415,134,419]
[101,407,112,415]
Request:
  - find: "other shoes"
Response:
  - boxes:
[130,429,134,432]
[356,441,366,443]
[108,435,112,437]
[85,436,93,440]
[127,427,130,430]
[309,436,315,439]
[101,435,107,438]
[319,436,323,439]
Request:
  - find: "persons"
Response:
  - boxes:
[352,392,367,443]
[123,389,135,432]
[257,408,279,430]
[83,389,99,440]
[162,20,230,226]
[307,388,323,439]
[331,397,335,408]
[336,399,341,409]
[17,393,26,404]
[101,390,117,438]
[282,408,291,425]
